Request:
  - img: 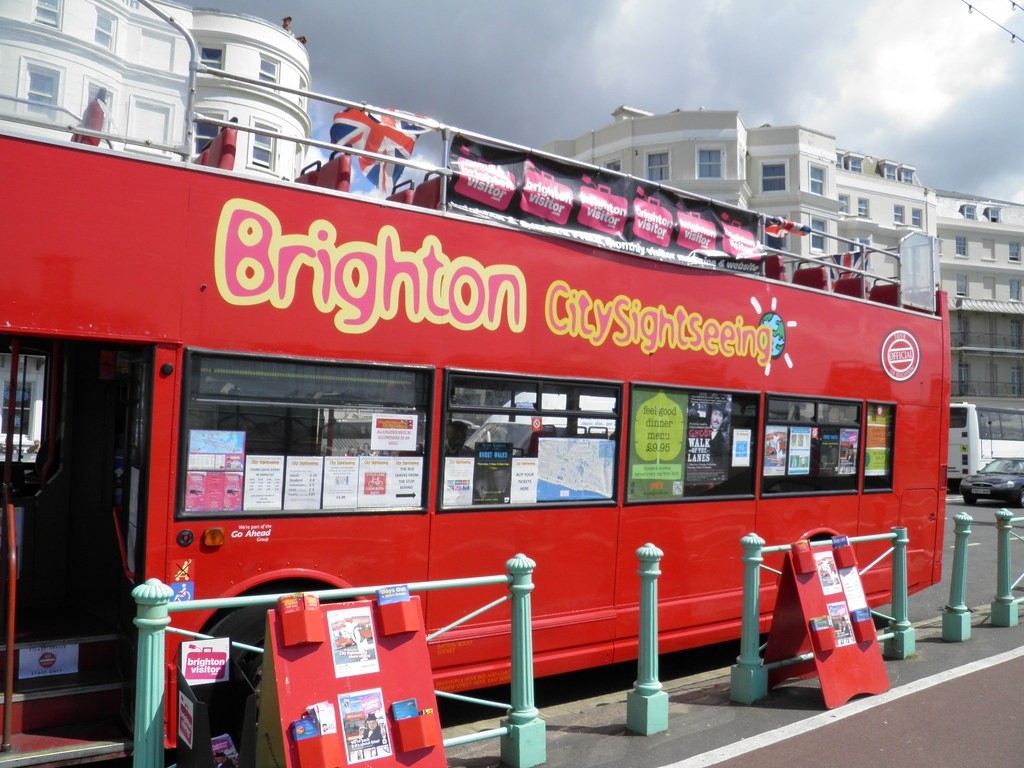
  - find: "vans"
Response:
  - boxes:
[463,392,618,504]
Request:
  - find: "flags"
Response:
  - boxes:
[830,254,869,293]
[330,101,438,195]
[765,217,811,239]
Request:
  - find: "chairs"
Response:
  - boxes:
[833,271,866,299]
[71,86,106,147]
[386,165,450,210]
[869,276,901,307]
[295,143,353,192]
[193,116,238,170]
[793,259,829,291]
[512,447,524,459]
[766,247,785,282]
[588,427,608,440]
[563,426,587,438]
[904,291,932,316]
[528,425,556,457]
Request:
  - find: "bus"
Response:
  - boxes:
[0,0,951,768]
[947,402,1024,493]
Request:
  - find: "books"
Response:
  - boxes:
[391,698,419,722]
[852,607,871,621]
[809,616,829,631]
[376,584,411,606]
[292,718,319,741]
[832,535,849,550]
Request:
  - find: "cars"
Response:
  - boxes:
[959,457,1024,508]
[318,420,398,456]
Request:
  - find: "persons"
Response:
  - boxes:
[688,394,729,458]
[772,435,786,465]
[214,752,236,768]
[846,446,854,466]
[445,421,474,458]
[359,714,388,747]
[822,560,839,585]
[322,724,328,732]
[343,621,375,661]
[345,443,398,456]
[839,617,851,638]
